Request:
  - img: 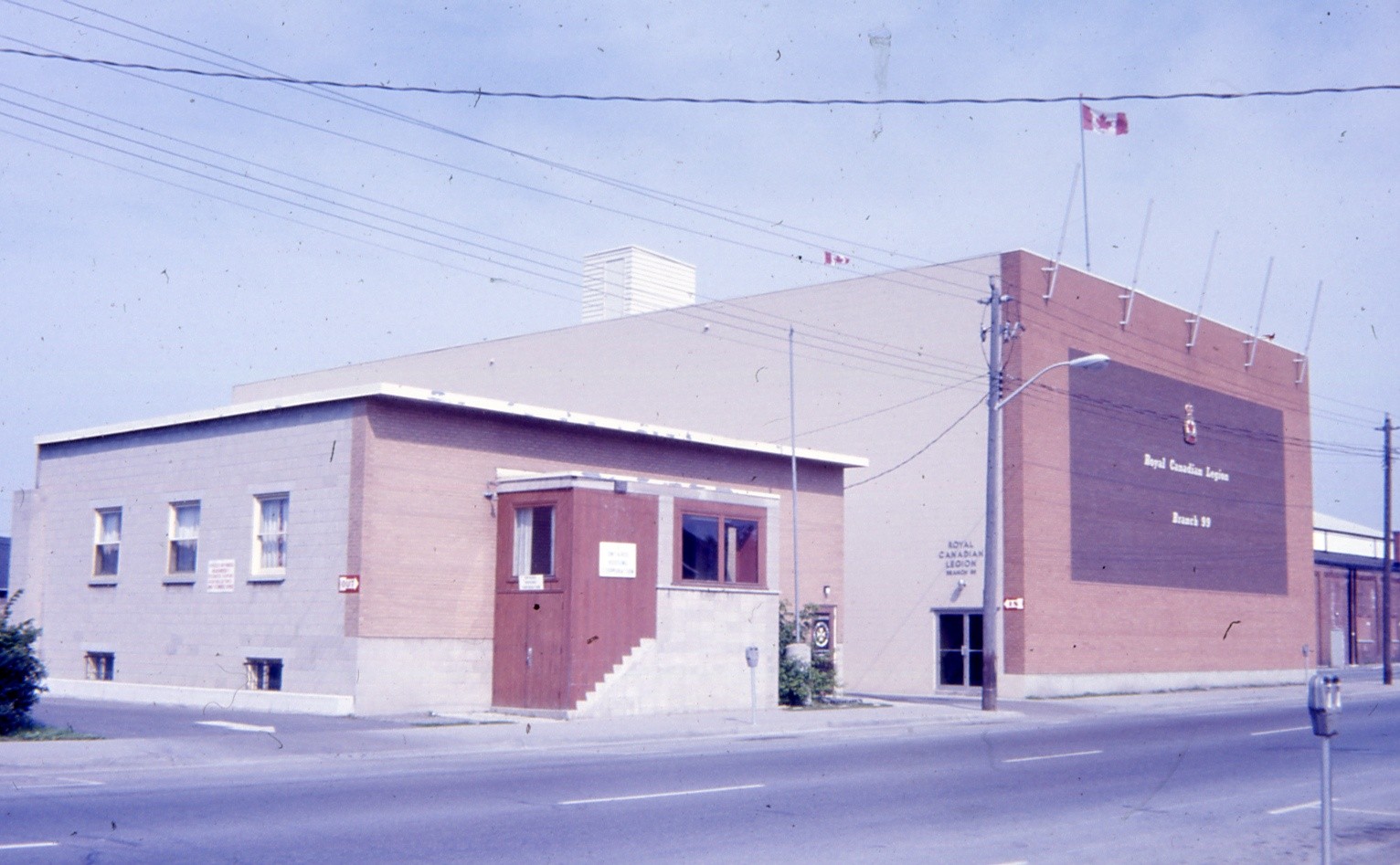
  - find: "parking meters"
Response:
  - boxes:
[1304,668,1347,864]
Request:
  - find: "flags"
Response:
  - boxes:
[1081,103,1129,135]
[824,251,850,265]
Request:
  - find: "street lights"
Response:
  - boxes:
[977,274,1114,710]
